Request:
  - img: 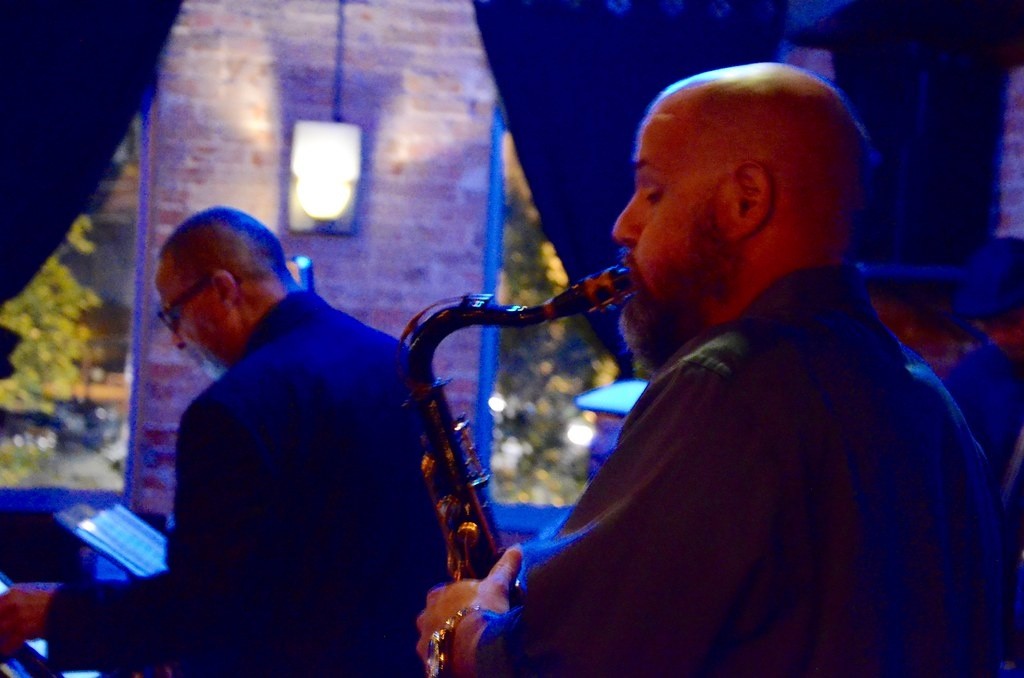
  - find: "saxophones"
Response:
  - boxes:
[401,263,638,601]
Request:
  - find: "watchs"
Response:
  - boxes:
[425,607,483,678]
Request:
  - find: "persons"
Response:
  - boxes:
[0,206,491,678]
[416,62,1024,678]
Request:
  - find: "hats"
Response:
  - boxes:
[953,236,1024,318]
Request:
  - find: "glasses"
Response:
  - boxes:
[157,275,243,331]
